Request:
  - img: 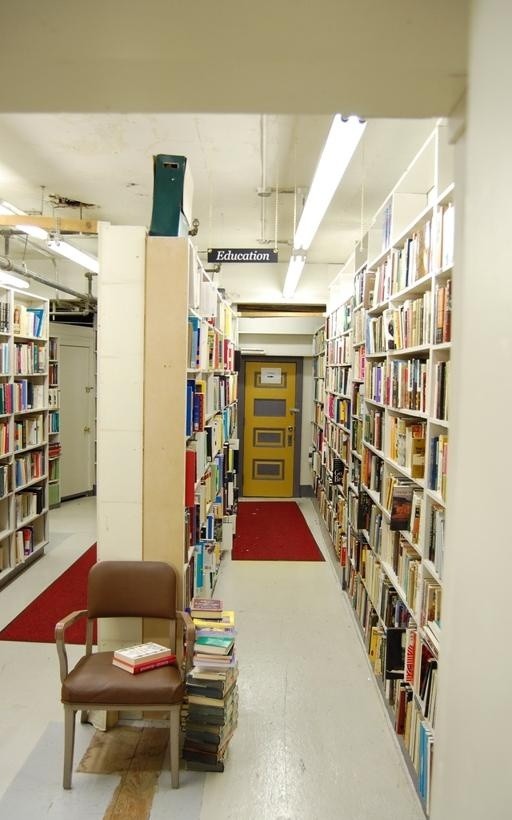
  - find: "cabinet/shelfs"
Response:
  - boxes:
[49,336,61,510]
[0,286,50,586]
[98,222,143,718]
[310,117,456,820]
[143,236,238,722]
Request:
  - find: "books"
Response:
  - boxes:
[352,419,365,455]
[319,491,326,518]
[355,541,374,594]
[0,523,35,573]
[331,516,347,566]
[364,409,428,477]
[364,221,430,310]
[0,449,45,497]
[187,375,237,441]
[427,502,443,581]
[214,483,238,550]
[182,598,237,774]
[185,481,218,565]
[49,337,56,359]
[187,427,219,488]
[425,434,447,505]
[430,359,448,422]
[342,559,363,610]
[190,245,219,329]
[352,384,365,417]
[349,456,362,495]
[364,448,385,505]
[326,297,352,340]
[370,506,393,564]
[323,446,344,486]
[431,279,451,344]
[325,367,352,394]
[327,392,351,430]
[312,469,322,502]
[49,387,60,412]
[326,417,349,460]
[0,341,45,375]
[385,473,425,545]
[370,564,398,629]
[395,600,419,630]
[393,531,422,613]
[359,588,374,637]
[48,412,59,433]
[352,309,366,345]
[112,642,178,674]
[219,301,236,346]
[218,442,234,493]
[188,316,234,373]
[419,578,442,630]
[49,457,58,484]
[314,356,324,377]
[0,487,45,533]
[363,358,428,414]
[429,203,453,273]
[364,290,431,354]
[315,378,323,403]
[48,439,60,458]
[347,489,376,530]
[352,346,365,382]
[395,678,432,800]
[314,327,324,354]
[383,626,438,717]
[0,379,45,415]
[308,445,322,478]
[350,531,367,563]
[312,423,323,452]
[0,302,44,338]
[323,476,346,527]
[314,401,324,426]
[185,543,219,613]
[0,414,45,456]
[327,332,353,364]
[354,266,369,307]
[365,614,397,706]
[48,362,58,387]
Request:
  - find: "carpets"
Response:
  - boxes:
[0,542,97,645]
[231,501,326,561]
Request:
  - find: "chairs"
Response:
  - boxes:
[55,560,196,789]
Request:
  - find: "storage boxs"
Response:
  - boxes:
[149,154,193,237]
